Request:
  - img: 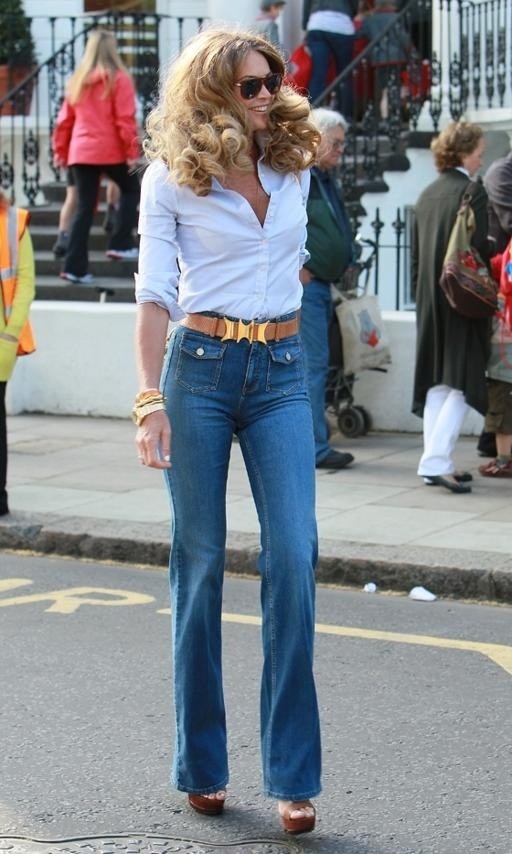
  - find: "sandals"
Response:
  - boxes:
[279,798,316,832]
[188,793,224,815]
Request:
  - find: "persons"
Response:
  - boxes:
[411,120,512,496]
[253,2,431,475]
[132,27,330,836]
[52,97,123,255]
[51,25,143,284]
[1,174,37,521]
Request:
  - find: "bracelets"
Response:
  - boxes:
[133,388,168,427]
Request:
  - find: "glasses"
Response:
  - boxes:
[330,139,348,151]
[232,71,282,100]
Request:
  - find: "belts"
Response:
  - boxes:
[180,315,299,344]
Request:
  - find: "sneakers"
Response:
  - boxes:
[60,272,93,283]
[478,460,511,477]
[106,247,139,260]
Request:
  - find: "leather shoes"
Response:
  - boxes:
[424,475,471,494]
[317,451,353,469]
[424,472,472,485]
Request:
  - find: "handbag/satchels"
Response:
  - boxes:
[334,285,392,376]
[439,184,498,321]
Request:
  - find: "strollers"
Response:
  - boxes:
[329,219,381,438]
